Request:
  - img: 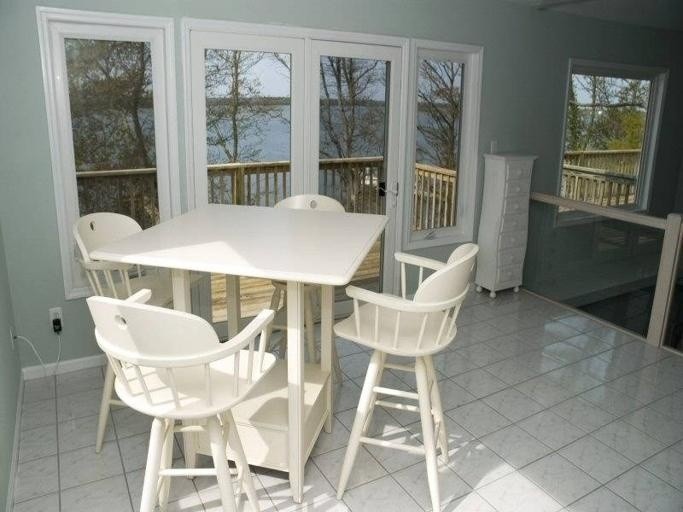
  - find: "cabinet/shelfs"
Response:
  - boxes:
[474,153,539,298]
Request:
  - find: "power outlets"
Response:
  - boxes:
[48,307,63,336]
